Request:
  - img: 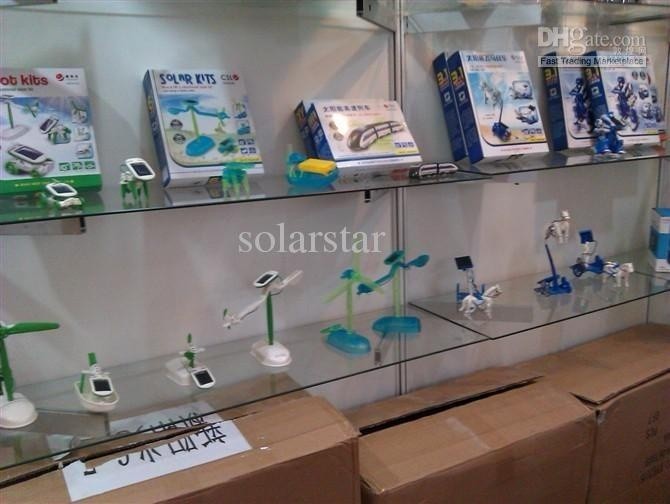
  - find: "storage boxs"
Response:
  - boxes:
[341,366,597,503]
[515,320,669,501]
[0,373,360,504]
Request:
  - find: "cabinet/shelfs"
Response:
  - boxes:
[0,3,670,470]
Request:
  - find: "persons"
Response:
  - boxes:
[479,80,513,142]
[222,161,255,200]
[286,145,339,191]
[570,229,635,287]
[593,112,627,157]
[347,121,404,151]
[169,100,231,156]
[455,256,503,320]
[408,162,459,179]
[534,209,571,297]
[569,78,595,134]
[612,77,639,132]
